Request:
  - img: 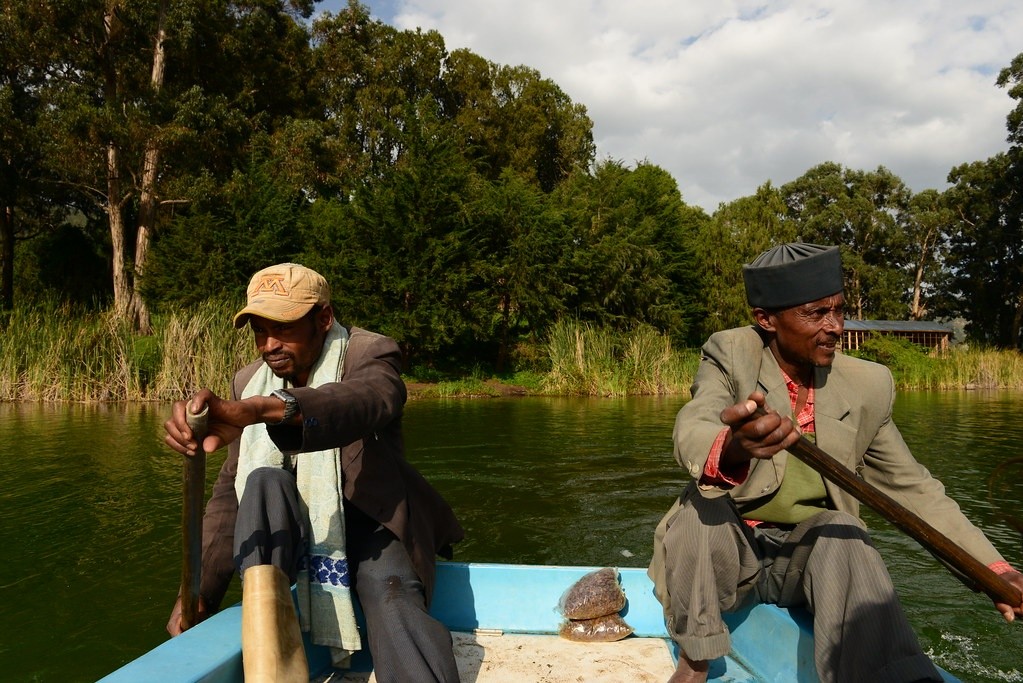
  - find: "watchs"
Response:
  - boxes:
[264,390,298,426]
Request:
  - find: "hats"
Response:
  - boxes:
[743,243,846,311]
[234,262,331,329]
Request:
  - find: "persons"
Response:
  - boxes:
[164,263,464,683]
[647,243,1023,682]
